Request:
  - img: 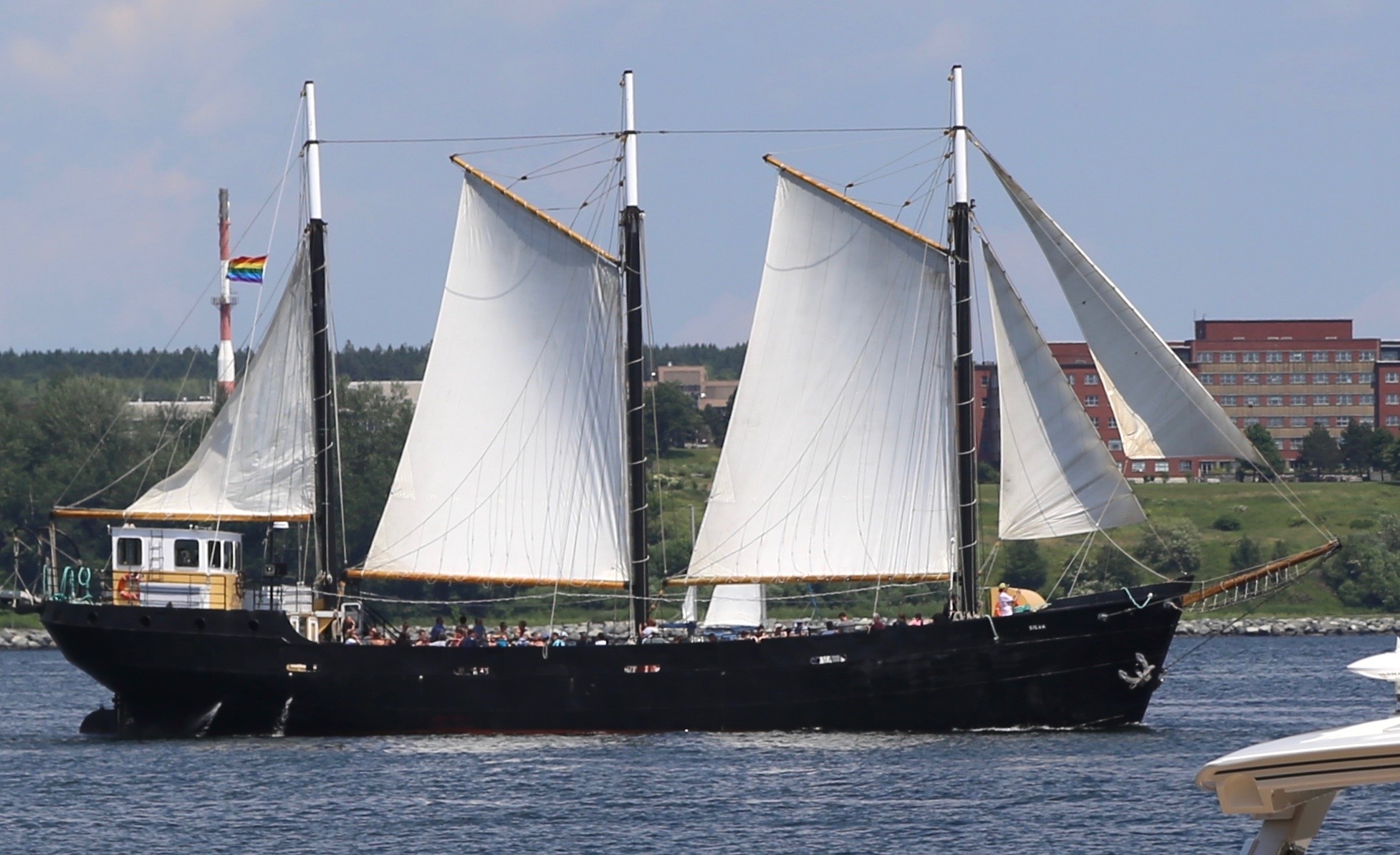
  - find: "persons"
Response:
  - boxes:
[342,612,919,647]
[998,582,1021,617]
[943,595,960,615]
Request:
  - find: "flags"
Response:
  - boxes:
[225,254,267,284]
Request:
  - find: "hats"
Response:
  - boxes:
[552,628,560,638]
[999,583,1010,590]
[562,632,568,638]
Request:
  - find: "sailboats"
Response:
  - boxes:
[39,62,1342,749]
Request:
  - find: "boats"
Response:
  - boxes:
[0,525,44,614]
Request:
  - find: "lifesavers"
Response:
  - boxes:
[118,573,144,600]
[995,600,1018,617]
